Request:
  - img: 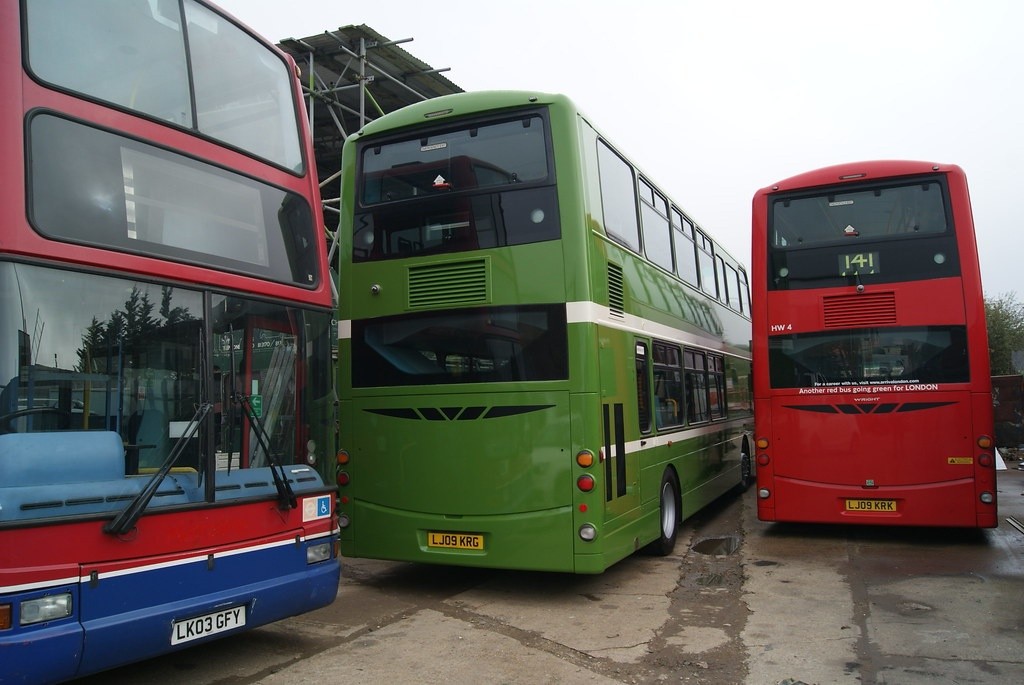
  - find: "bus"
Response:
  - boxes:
[0,0,340,685]
[752,161,999,529]
[337,91,751,576]
